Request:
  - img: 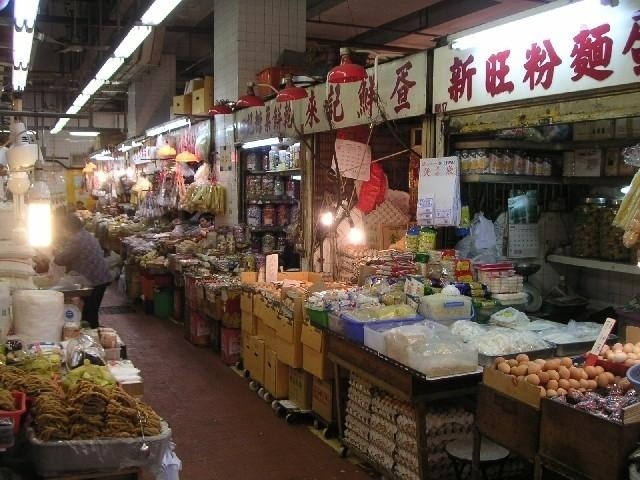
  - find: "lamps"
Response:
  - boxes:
[341,203,365,245]
[176,124,197,162]
[157,124,176,155]
[320,203,341,227]
[24,115,53,247]
[11,0,41,92]
[208,47,389,115]
[447,0,619,51]
[49,0,183,135]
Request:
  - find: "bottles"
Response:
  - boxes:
[455,147,552,177]
[576,197,629,260]
[246,146,279,170]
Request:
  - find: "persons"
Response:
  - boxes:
[198,212,214,231]
[53,215,113,328]
[77,201,86,210]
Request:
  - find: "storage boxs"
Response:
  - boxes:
[192,76,214,116]
[240,271,472,423]
[173,95,192,114]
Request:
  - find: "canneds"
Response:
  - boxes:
[457,148,556,177]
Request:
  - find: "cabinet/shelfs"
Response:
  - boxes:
[453,117,640,277]
[241,141,301,271]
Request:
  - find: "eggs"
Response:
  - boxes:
[491,348,623,398]
[376,392,416,418]
[598,340,640,367]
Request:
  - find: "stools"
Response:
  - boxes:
[445,439,511,480]
